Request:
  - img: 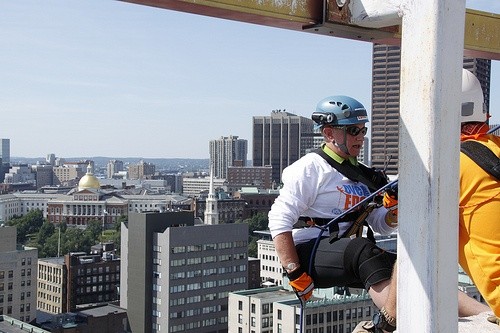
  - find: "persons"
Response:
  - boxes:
[268,95,490,317]
[352,69,500,333]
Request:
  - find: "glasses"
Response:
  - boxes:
[331,125,368,136]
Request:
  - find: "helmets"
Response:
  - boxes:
[312,95,369,130]
[461,68,487,124]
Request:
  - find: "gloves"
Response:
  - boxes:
[352,321,380,333]
[286,266,314,302]
[383,190,397,216]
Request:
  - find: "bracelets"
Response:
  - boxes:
[284,260,299,271]
